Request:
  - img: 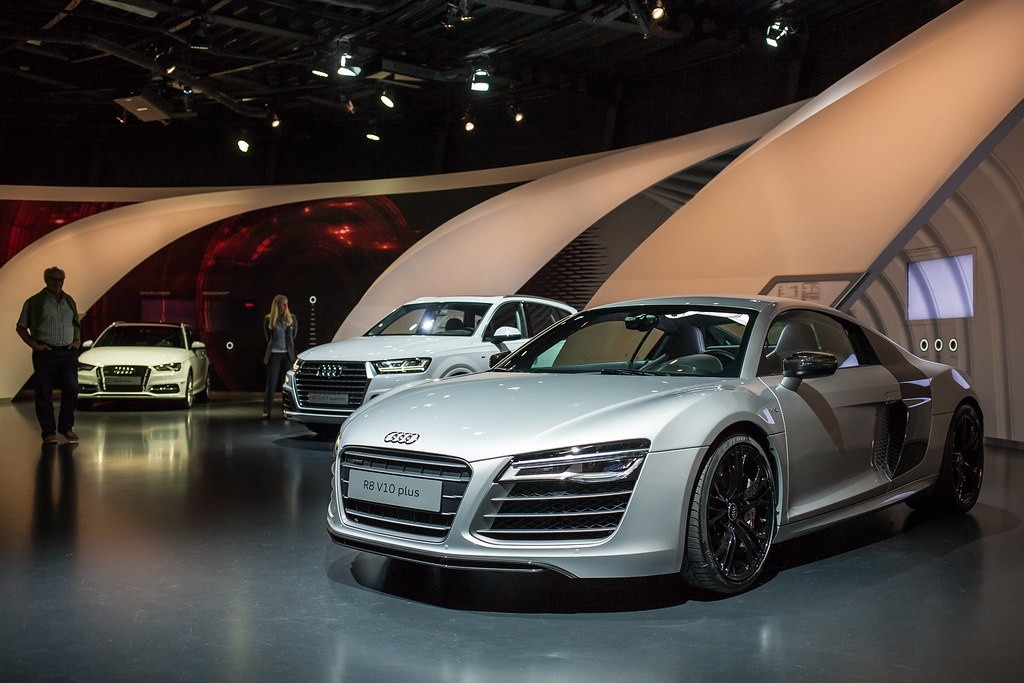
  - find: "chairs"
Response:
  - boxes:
[648,324,706,364]
[477,319,496,336]
[445,319,463,331]
[764,322,822,374]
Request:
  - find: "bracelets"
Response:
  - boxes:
[75,339,81,342]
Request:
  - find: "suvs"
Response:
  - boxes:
[71,319,213,412]
[282,294,576,436]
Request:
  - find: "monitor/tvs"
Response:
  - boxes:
[906,247,977,325]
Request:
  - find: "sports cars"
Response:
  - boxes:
[326,294,986,595]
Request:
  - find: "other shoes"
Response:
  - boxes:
[261,412,271,417]
[42,434,58,444]
[58,429,79,442]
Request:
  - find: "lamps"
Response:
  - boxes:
[270,112,282,128]
[765,13,790,48]
[440,0,475,29]
[309,34,362,79]
[642,0,669,33]
[466,42,495,93]
[362,117,384,144]
[152,44,177,75]
[463,112,480,134]
[506,103,526,124]
[382,85,403,108]
[183,85,195,111]
[236,129,259,155]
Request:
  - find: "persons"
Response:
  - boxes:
[16,267,81,445]
[262,295,298,421]
